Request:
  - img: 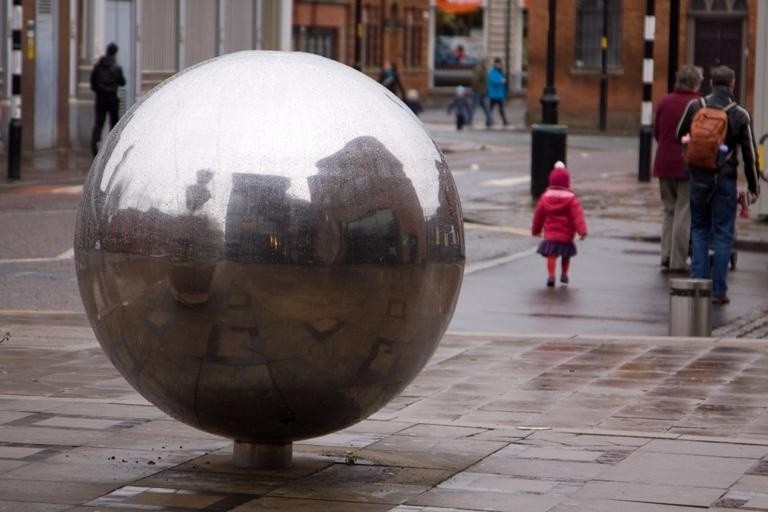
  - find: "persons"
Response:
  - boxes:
[377,53,511,131]
[651,64,762,303]
[530,160,588,285]
[88,41,127,159]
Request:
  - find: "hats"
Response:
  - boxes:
[548,159,571,188]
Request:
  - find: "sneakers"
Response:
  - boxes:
[561,276,570,284]
[548,279,554,289]
[713,295,730,306]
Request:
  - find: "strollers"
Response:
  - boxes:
[688,188,749,271]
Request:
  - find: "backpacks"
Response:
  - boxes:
[680,97,737,172]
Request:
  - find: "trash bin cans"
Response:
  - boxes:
[670,278,713,337]
